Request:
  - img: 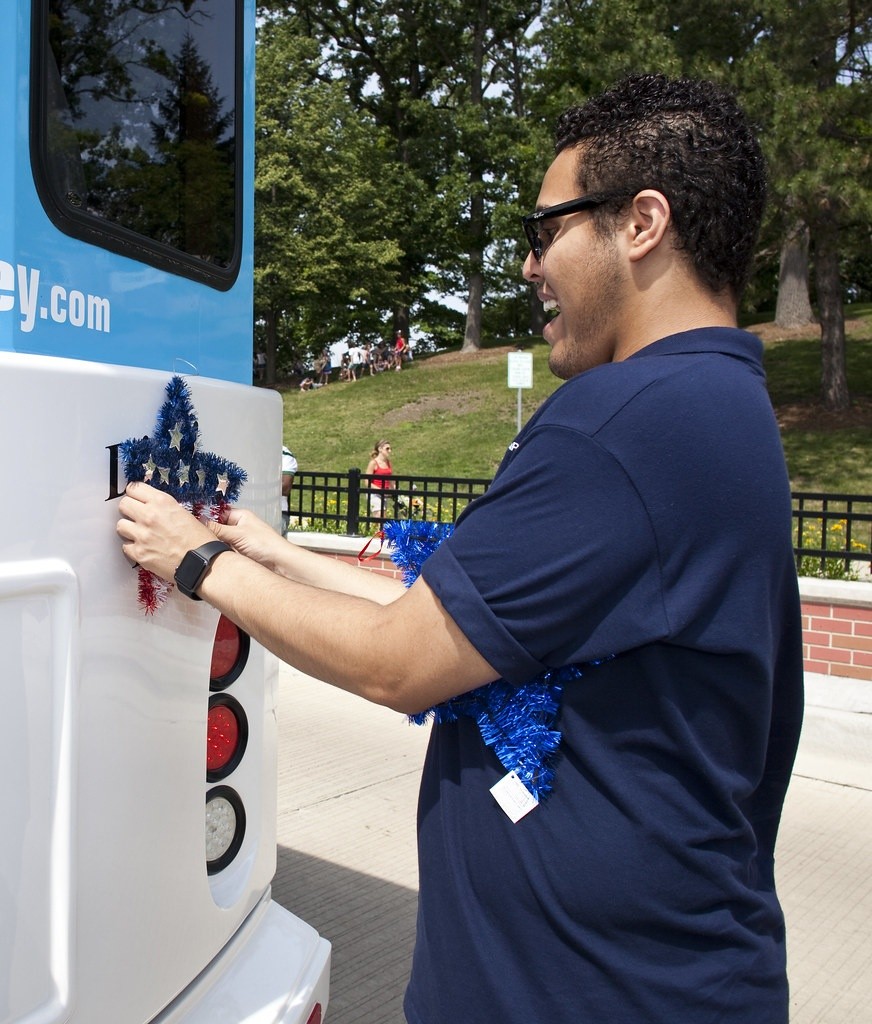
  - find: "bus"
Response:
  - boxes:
[3,1,334,1018]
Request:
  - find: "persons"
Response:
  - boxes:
[117,69,804,1024]
[253,348,268,382]
[364,440,396,531]
[282,443,298,541]
[291,330,406,392]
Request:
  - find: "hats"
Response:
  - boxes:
[396,329,403,335]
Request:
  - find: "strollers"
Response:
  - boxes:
[384,493,434,522]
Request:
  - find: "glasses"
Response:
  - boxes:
[522,187,681,263]
[382,447,392,451]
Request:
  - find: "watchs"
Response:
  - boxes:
[174,541,236,601]
